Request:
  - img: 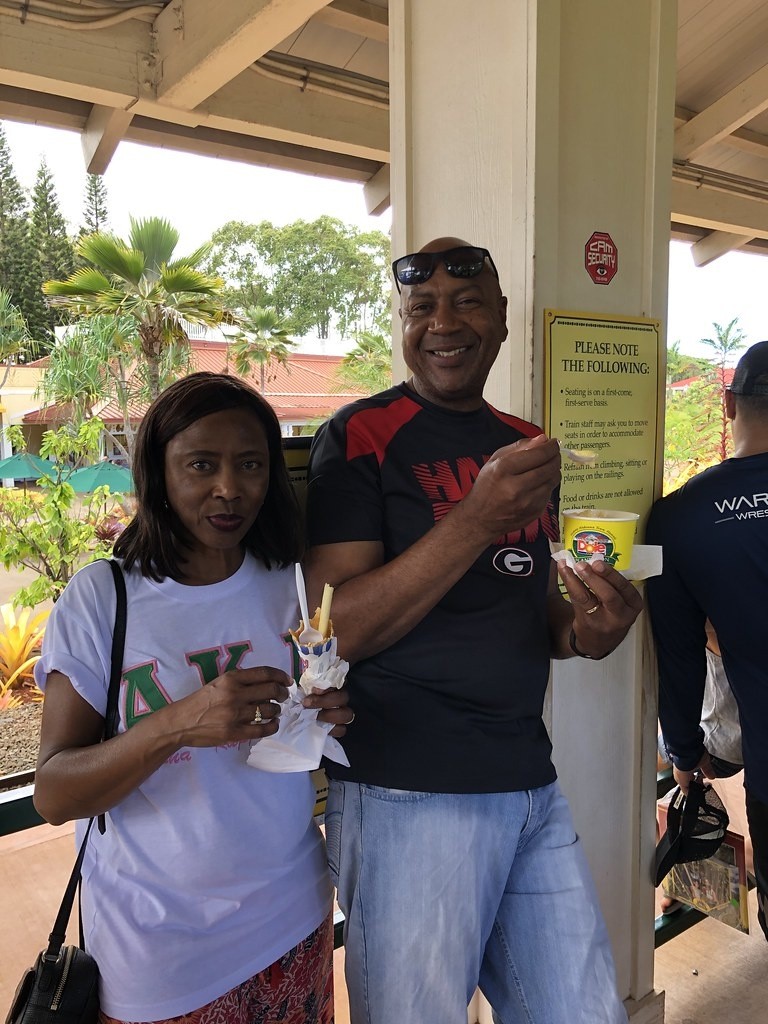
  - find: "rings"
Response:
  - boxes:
[345,712,354,724]
[254,707,262,723]
[586,604,600,614]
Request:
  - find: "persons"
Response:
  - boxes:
[302,238,644,1024]
[639,338,768,946]
[683,859,718,909]
[106,511,127,533]
[655,615,746,916]
[31,372,355,1023]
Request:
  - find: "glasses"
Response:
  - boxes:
[391,246,499,301]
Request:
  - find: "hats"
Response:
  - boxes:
[653,779,730,887]
[731,340,768,396]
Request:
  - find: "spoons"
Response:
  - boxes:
[295,562,323,644]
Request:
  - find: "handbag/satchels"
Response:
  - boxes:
[5,943,102,1024]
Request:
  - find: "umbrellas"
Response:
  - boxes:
[39,461,136,521]
[0,451,72,521]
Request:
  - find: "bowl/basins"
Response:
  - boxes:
[562,508,640,571]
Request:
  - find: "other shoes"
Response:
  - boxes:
[663,897,685,916]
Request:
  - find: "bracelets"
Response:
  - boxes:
[570,629,615,660]
